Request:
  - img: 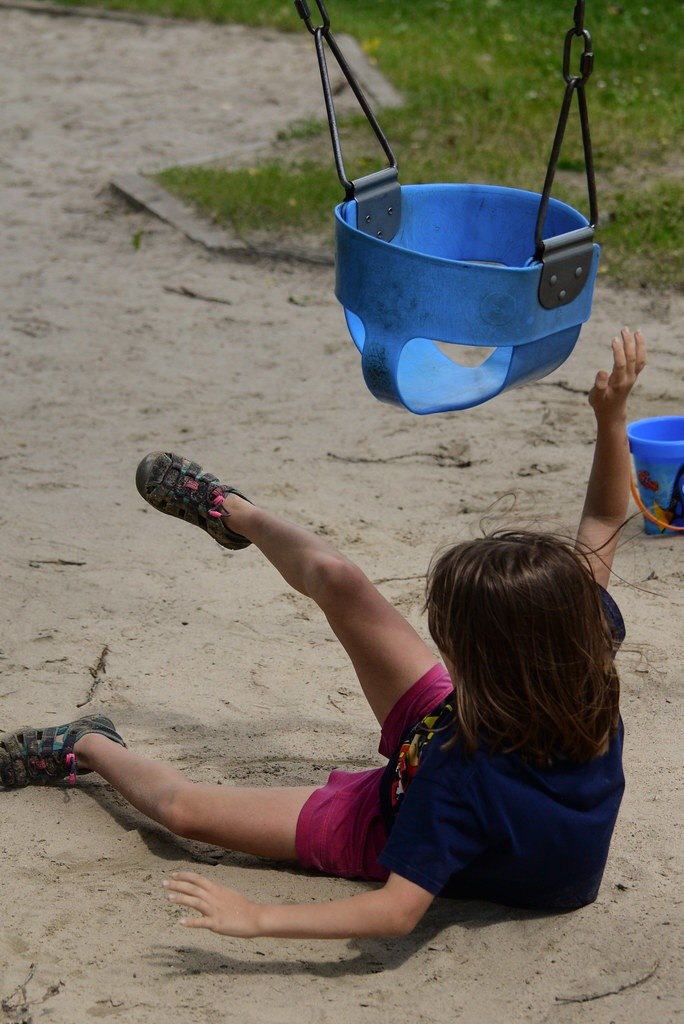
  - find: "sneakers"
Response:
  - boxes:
[134,448,256,554]
[0,711,126,798]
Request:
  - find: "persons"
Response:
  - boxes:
[1,328,648,941]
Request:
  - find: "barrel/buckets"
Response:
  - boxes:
[624,415,684,536]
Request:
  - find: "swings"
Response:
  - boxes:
[292,0,602,417]
